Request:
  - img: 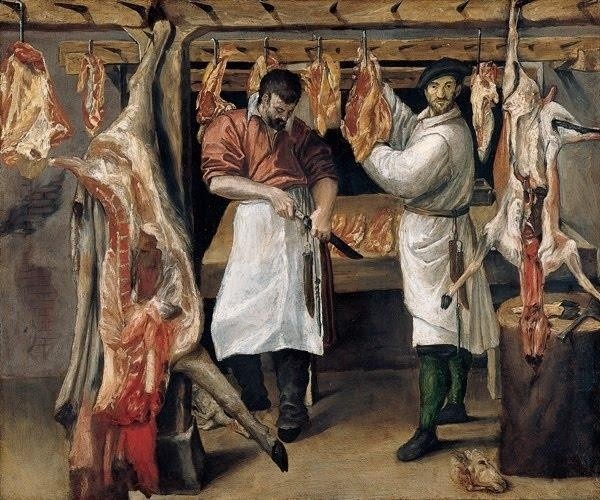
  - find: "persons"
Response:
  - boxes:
[349,53,500,461]
[199,69,340,442]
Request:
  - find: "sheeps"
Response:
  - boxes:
[440,0,600,371]
[448,445,508,494]
[45,18,289,500]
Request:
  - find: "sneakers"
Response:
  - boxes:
[242,397,273,411]
[433,404,469,426]
[397,426,438,461]
[277,414,310,442]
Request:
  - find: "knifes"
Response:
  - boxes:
[292,208,364,260]
[557,297,600,342]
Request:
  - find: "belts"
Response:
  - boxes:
[402,204,470,219]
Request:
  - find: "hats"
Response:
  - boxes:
[416,57,467,91]
